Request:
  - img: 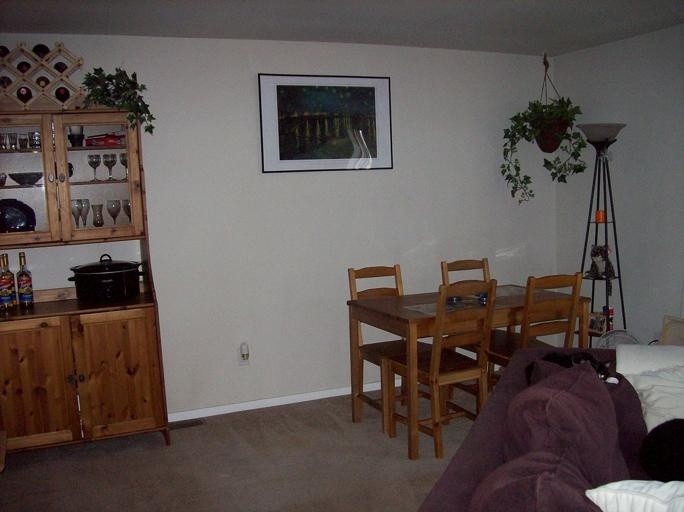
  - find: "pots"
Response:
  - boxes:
[68,253,148,304]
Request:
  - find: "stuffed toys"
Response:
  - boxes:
[584,243,616,281]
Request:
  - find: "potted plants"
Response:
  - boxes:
[500,97,587,204]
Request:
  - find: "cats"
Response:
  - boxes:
[524,350,623,387]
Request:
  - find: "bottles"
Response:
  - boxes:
[1,42,70,103]
[1,251,34,315]
[278,108,377,153]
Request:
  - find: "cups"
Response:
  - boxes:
[595,210,605,223]
[0,131,41,149]
[67,133,85,146]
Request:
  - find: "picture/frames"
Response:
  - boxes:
[257,73,393,173]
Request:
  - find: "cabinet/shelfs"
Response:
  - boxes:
[0,109,145,249]
[0,303,169,472]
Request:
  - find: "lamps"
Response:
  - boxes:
[240,344,249,360]
[576,122,627,348]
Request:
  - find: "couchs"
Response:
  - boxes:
[418,346,648,512]
[617,344,684,433]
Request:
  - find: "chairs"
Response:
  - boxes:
[389,278,496,457]
[478,272,582,367]
[348,264,430,432]
[441,258,520,400]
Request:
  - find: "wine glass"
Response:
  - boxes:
[71,196,131,229]
[87,152,128,181]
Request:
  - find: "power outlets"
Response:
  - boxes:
[238,350,248,365]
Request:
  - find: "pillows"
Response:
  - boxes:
[585,479,684,512]
[469,452,603,512]
[637,418,684,482]
[500,360,628,487]
[530,360,565,387]
[526,352,606,383]
[608,373,646,479]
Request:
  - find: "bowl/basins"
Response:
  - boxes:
[8,172,44,185]
